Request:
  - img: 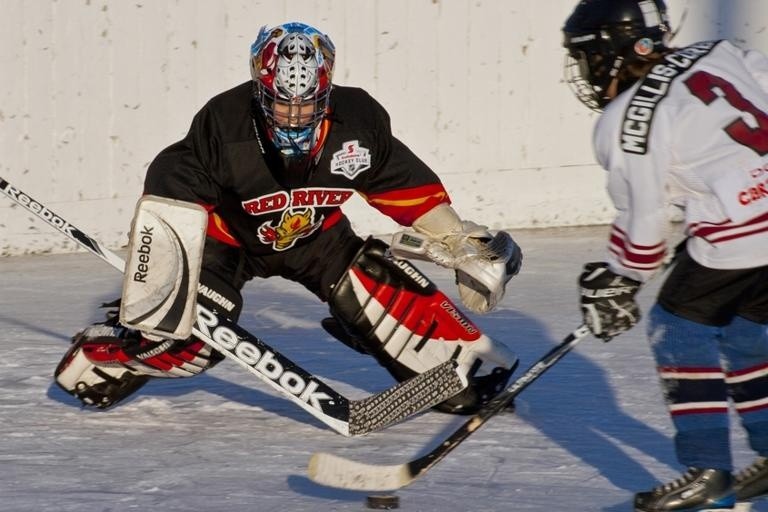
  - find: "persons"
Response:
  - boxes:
[54,19,525,418]
[560,0,768,512]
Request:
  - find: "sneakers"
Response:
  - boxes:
[735,455,768,503]
[636,466,737,512]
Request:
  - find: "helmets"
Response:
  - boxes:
[561,0,675,113]
[249,20,336,159]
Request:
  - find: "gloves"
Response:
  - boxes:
[579,261,642,342]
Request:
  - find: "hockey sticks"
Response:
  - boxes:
[305,255,676,491]
[0,179,469,439]
